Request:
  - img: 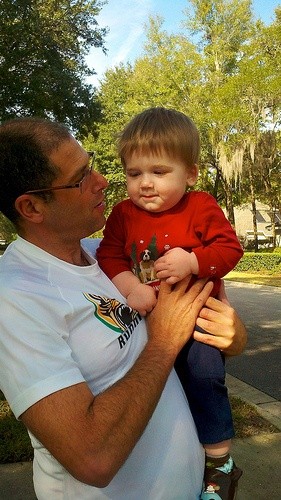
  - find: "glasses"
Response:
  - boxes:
[24,151,96,194]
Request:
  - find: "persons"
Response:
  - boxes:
[0,116,249,500]
[95,106,244,500]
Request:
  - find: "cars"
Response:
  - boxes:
[245,229,270,249]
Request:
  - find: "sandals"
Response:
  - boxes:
[200,455,243,500]
